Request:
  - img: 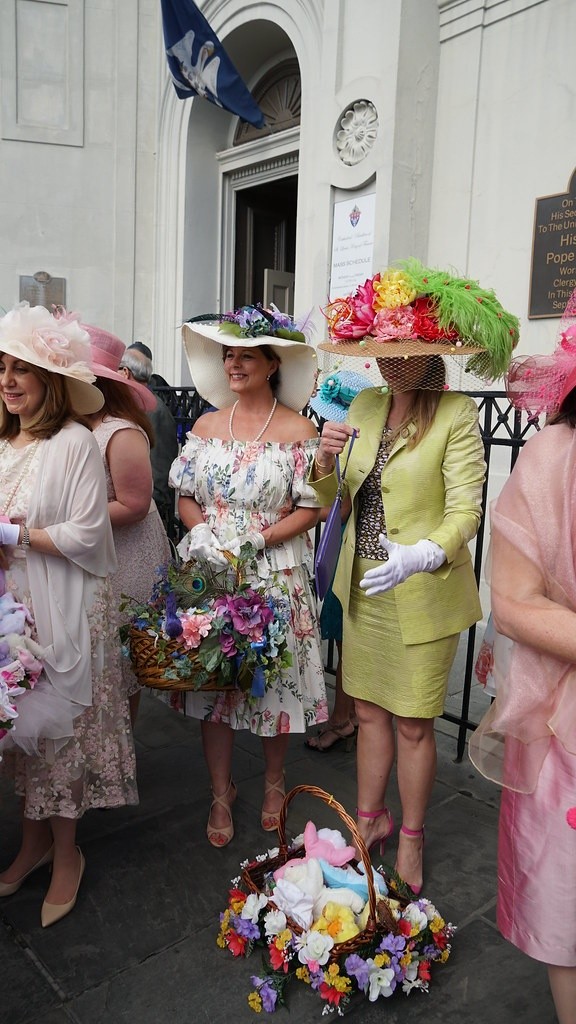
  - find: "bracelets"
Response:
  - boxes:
[315,455,336,468]
[315,462,334,475]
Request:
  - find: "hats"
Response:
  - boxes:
[506,289,576,421]
[77,304,159,412]
[316,256,520,384]
[309,372,375,424]
[182,302,318,413]
[127,342,152,361]
[0,300,105,415]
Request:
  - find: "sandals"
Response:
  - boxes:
[260,768,286,831]
[206,773,237,848]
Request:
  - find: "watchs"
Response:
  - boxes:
[21,528,30,550]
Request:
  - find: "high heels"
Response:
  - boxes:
[0,841,54,897]
[349,805,394,859]
[317,712,359,747]
[394,824,425,897]
[41,845,86,929]
[304,720,356,754]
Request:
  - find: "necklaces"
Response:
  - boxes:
[229,396,277,443]
[0,434,40,516]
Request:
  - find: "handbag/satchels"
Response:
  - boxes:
[315,429,357,602]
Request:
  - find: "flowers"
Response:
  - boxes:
[216,833,458,1017]
[317,375,355,411]
[1,299,97,383]
[219,303,306,344]
[119,529,293,706]
[0,649,43,739]
[318,272,459,343]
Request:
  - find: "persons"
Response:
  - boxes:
[0,298,184,928]
[303,358,488,896]
[167,321,379,848]
[465,290,576,1024]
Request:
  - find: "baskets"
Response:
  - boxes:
[128,549,244,691]
[242,785,414,975]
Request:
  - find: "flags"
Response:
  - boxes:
[161,0,265,131]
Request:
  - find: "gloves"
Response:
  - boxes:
[359,534,447,597]
[221,532,265,566]
[188,524,229,569]
[0,523,19,546]
